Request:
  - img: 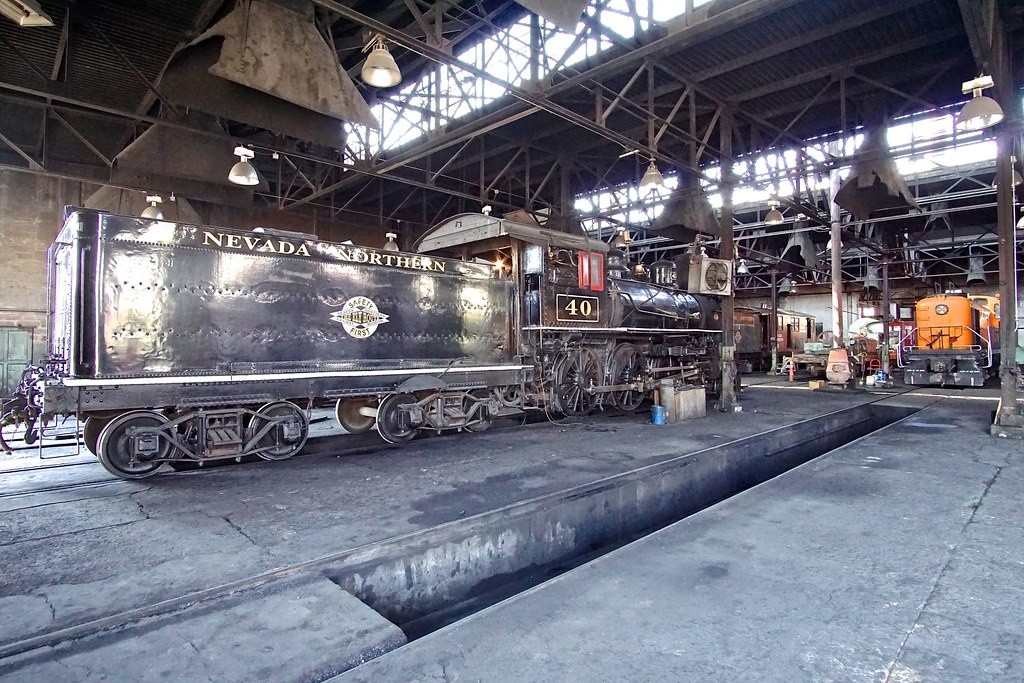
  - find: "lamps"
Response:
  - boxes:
[826,230,843,248]
[789,281,800,295]
[991,154,1022,188]
[140,195,165,219]
[227,147,259,186]
[640,159,664,190]
[362,36,402,88]
[737,259,749,274]
[764,200,784,224]
[382,233,400,252]
[1016,206,1024,228]
[953,75,1006,129]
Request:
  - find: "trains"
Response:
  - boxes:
[732,307,820,374]
[36,204,743,481]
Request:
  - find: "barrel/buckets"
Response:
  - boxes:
[651,405,666,426]
[877,371,886,382]
[872,375,878,381]
[866,376,875,385]
